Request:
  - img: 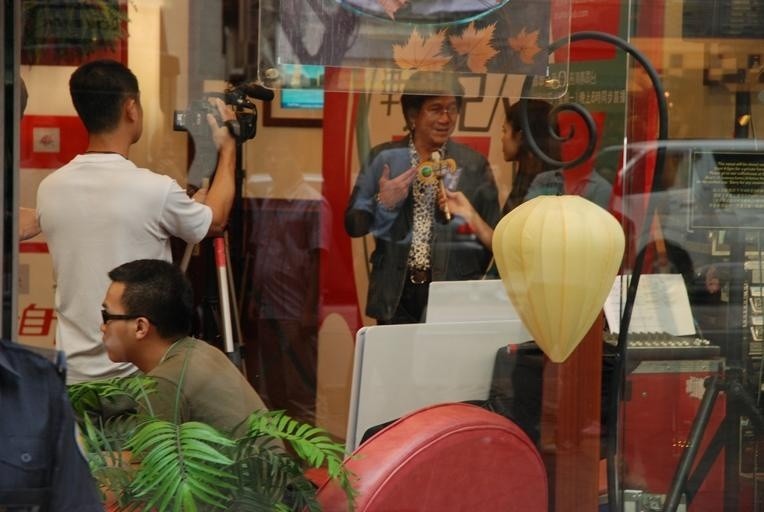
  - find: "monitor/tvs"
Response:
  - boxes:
[269,62,325,120]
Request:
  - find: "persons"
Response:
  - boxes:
[521,156,616,208]
[65,257,290,509]
[0,340,106,511]
[341,71,499,325]
[34,59,245,391]
[434,98,565,281]
[143,122,201,196]
[243,123,332,461]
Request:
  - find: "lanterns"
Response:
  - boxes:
[490,194,626,363]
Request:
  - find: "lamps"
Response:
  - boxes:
[490,30,669,511]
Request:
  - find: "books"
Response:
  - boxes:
[600,273,697,337]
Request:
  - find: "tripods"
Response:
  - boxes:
[178,231,246,375]
[662,241,764,512]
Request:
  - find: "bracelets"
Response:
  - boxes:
[377,192,382,204]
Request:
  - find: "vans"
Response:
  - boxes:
[589,136,763,302]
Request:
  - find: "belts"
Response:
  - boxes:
[406,268,432,285]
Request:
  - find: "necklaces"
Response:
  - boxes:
[83,150,126,159]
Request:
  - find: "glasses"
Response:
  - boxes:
[424,107,457,117]
[100,308,152,326]
